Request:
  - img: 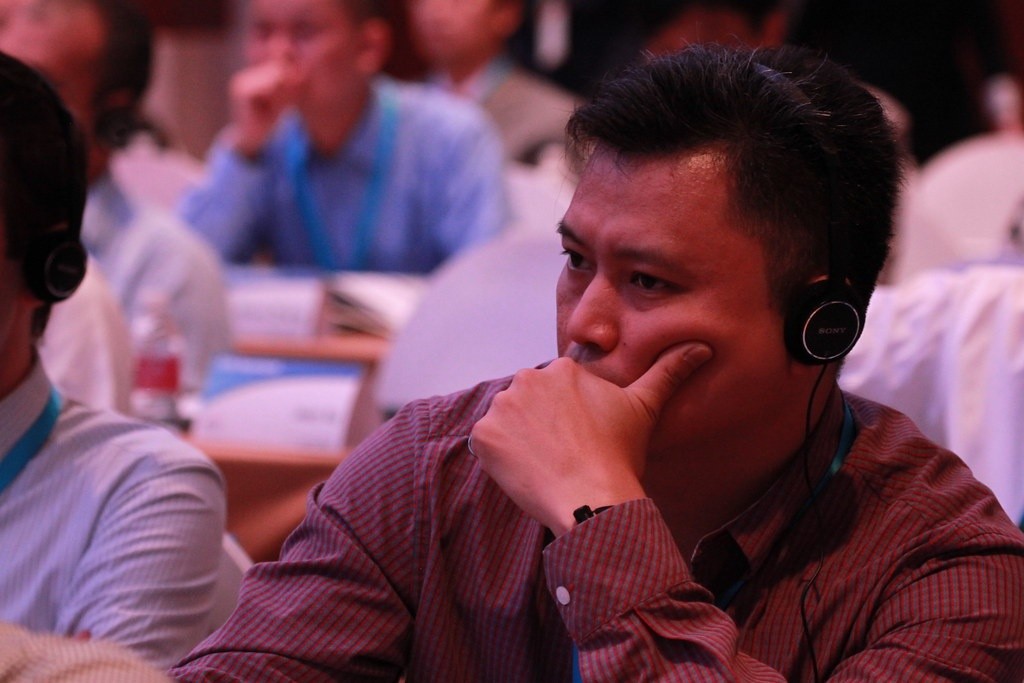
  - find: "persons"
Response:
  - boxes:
[0,1,1024,682]
[168,43,1024,683]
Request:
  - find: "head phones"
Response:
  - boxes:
[29,223,88,304]
[754,63,868,364]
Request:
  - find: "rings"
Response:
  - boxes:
[467,434,480,459]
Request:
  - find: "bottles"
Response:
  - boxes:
[130,289,195,426]
